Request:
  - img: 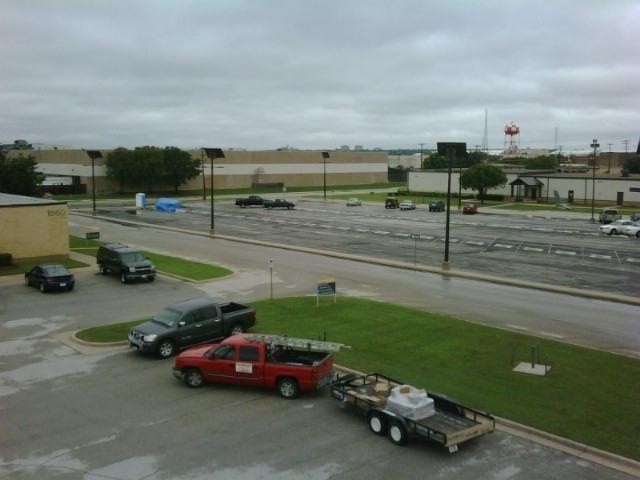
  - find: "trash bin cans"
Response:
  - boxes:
[617,192,623,204]
[568,190,574,203]
[136,193,145,209]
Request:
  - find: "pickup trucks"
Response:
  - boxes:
[235,195,296,210]
[128,297,334,399]
[96,244,155,283]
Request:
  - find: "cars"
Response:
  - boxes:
[346,198,361,206]
[385,197,416,210]
[463,203,477,214]
[599,208,640,238]
[24,263,75,294]
[429,199,445,212]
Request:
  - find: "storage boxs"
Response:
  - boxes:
[347,381,390,410]
